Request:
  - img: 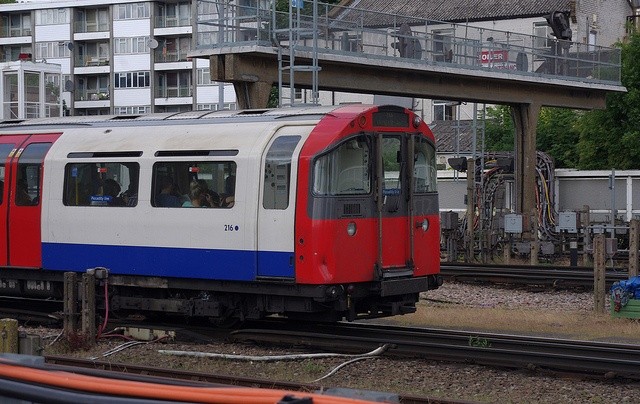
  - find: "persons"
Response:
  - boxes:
[64,166,138,206]
[199,177,221,208]
[16,178,32,204]
[156,176,185,207]
[218,175,235,208]
[181,179,217,207]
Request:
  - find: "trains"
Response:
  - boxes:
[0,105,443,329]
